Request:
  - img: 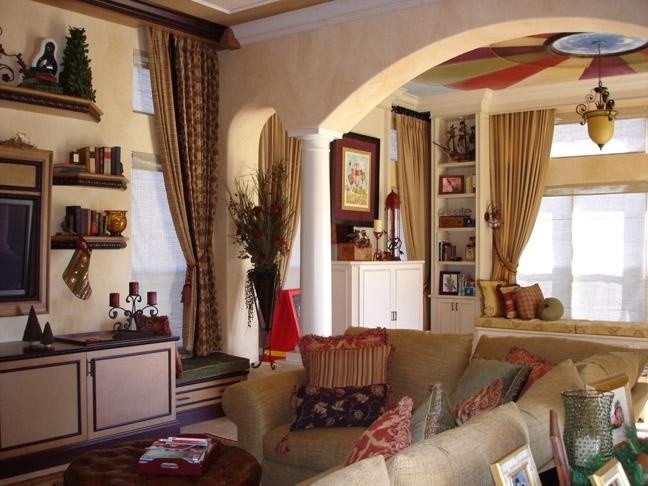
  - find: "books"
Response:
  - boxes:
[79,146,121,174]
[62,206,108,236]
[54,162,87,174]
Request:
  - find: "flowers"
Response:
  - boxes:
[224,159,296,333]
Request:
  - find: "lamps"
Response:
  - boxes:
[385,189,401,261]
[574,41,619,152]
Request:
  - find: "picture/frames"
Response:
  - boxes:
[0,147,54,318]
[587,373,635,448]
[588,457,631,486]
[550,409,572,486]
[490,442,541,486]
[439,174,464,194]
[440,271,460,295]
[331,131,380,226]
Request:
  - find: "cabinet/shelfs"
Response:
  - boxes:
[427,294,478,334]
[86,336,180,447]
[431,114,492,296]
[0,340,86,479]
[331,260,425,336]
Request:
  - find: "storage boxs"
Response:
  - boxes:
[337,243,372,261]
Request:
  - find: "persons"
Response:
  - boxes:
[610,401,624,429]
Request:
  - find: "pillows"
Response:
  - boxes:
[496,284,520,319]
[512,283,544,320]
[276,382,391,454]
[451,358,532,407]
[304,343,394,388]
[478,279,508,318]
[502,344,556,402]
[343,395,414,468]
[454,377,501,427]
[409,382,453,442]
[297,326,386,369]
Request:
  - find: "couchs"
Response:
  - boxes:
[221,326,648,486]
[64,432,262,486]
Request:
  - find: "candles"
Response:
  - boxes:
[110,293,119,305]
[129,281,139,295]
[373,219,382,232]
[147,292,157,305]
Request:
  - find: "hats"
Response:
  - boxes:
[614,400,623,415]
